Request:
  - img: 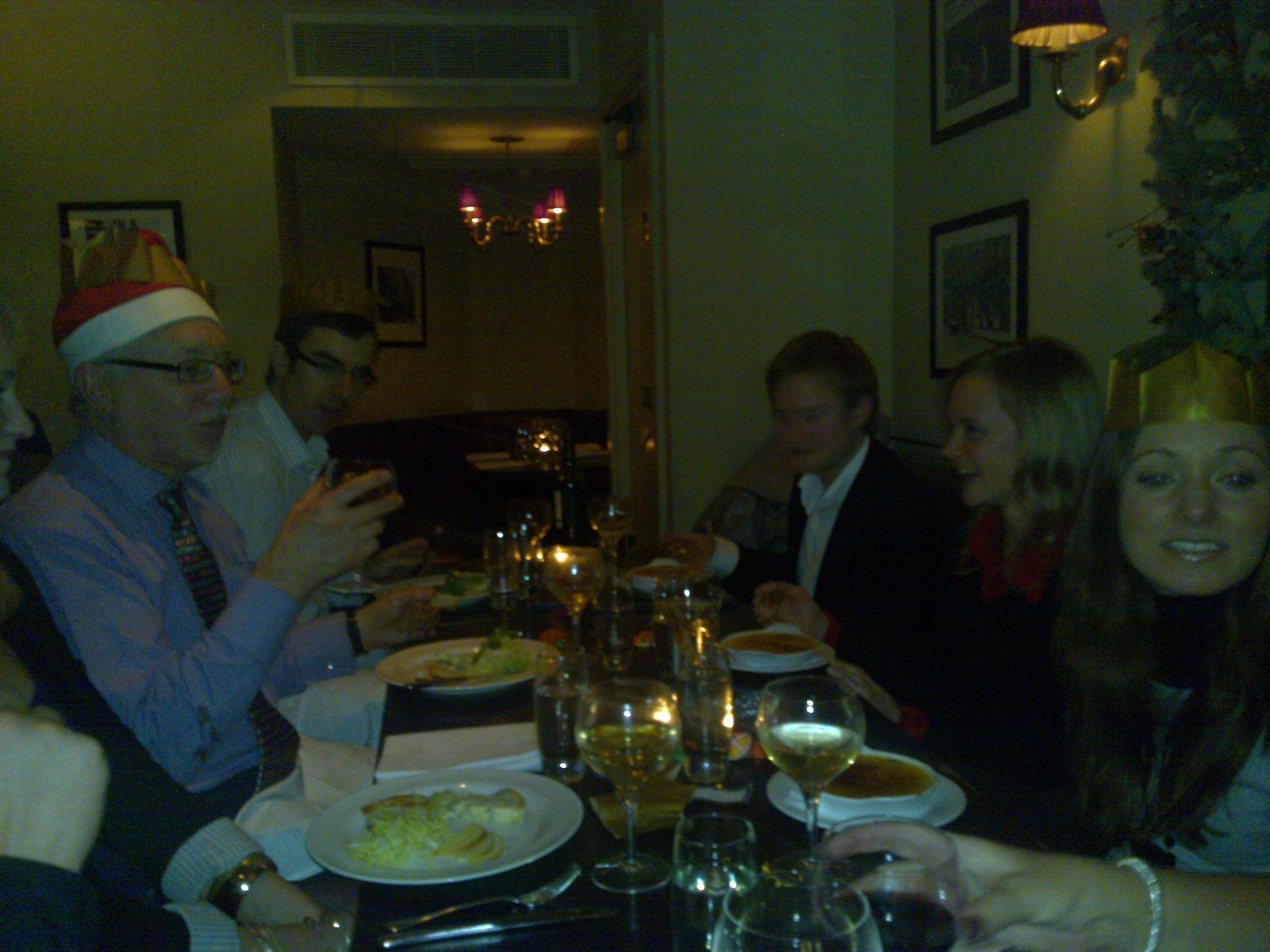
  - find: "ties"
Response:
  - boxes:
[158,485,301,801]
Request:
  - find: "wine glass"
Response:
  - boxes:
[316,460,395,596]
[574,680,682,893]
[756,676,866,852]
[482,501,725,678]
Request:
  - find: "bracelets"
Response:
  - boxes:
[345,608,368,657]
[1115,856,1161,952]
[213,851,277,918]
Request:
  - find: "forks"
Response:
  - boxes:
[383,868,576,932]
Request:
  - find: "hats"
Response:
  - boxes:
[51,227,226,388]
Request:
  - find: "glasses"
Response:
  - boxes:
[275,338,378,390]
[97,357,248,388]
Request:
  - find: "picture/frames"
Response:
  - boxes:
[360,239,429,349]
[927,198,1029,378]
[58,198,187,285]
[928,1,1031,147]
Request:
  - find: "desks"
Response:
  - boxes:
[348,567,957,951]
[469,443,608,530]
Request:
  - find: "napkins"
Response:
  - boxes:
[374,722,543,780]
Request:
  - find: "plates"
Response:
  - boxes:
[721,631,834,673]
[303,767,584,885]
[766,753,967,835]
[389,574,492,606]
[374,635,560,694]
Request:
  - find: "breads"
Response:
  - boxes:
[465,787,527,824]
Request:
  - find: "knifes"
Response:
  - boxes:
[379,912,608,948]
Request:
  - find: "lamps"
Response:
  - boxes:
[461,135,569,248]
[1012,0,1129,121]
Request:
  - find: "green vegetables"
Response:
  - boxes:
[469,624,519,668]
[431,570,482,596]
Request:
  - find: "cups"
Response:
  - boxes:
[711,868,882,951]
[813,814,972,952]
[669,817,756,952]
[678,674,734,781]
[534,644,592,781]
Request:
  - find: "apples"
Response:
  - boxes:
[432,825,504,864]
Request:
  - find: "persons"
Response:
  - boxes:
[630,329,1270,952]
[0,229,448,952]
